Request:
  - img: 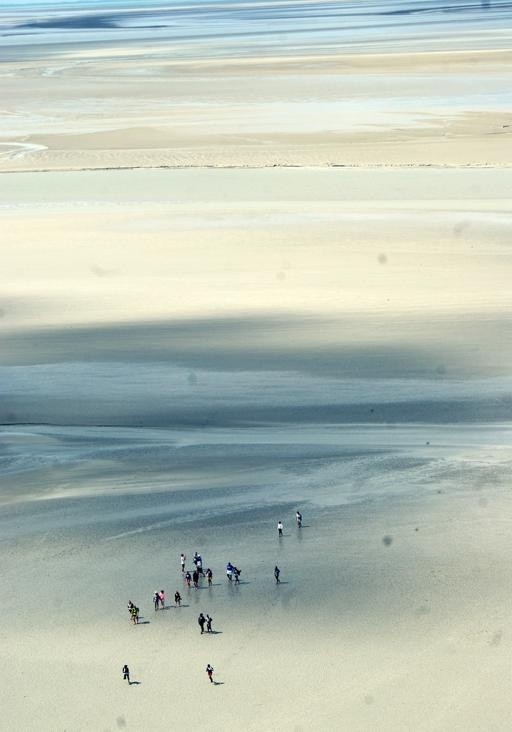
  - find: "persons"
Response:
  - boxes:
[159,589,165,609]
[206,663,214,682]
[277,520,283,536]
[206,568,213,586]
[153,591,160,610]
[121,664,130,682]
[195,557,206,578]
[198,612,206,634]
[128,599,133,620]
[185,570,192,586]
[274,565,280,585]
[193,551,201,566]
[225,560,234,581]
[131,604,140,626]
[181,553,187,573]
[192,570,199,590]
[174,589,182,608]
[296,511,303,528]
[233,566,242,585]
[206,613,213,632]
